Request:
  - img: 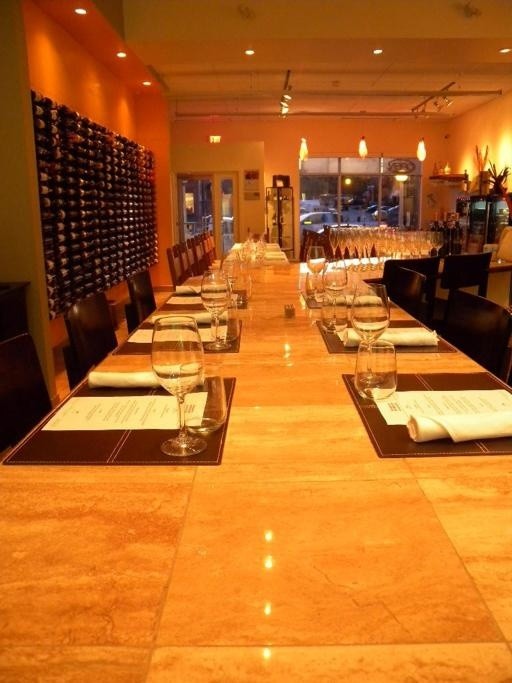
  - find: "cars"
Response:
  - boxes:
[299,193,400,238]
[208,217,233,252]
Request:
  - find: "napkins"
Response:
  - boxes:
[85,369,213,390]
[405,412,512,443]
[150,309,229,323]
[332,295,391,304]
[176,284,233,295]
[342,328,439,347]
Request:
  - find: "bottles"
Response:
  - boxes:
[33,91,158,319]
[433,160,450,176]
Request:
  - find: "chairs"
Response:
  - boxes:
[165,228,217,288]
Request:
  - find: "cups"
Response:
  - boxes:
[355,340,397,402]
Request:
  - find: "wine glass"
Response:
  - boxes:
[353,284,387,384]
[202,269,232,350]
[324,270,347,332]
[307,246,326,291]
[226,265,251,333]
[152,315,207,457]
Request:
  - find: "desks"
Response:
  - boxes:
[4,158,512,683]
